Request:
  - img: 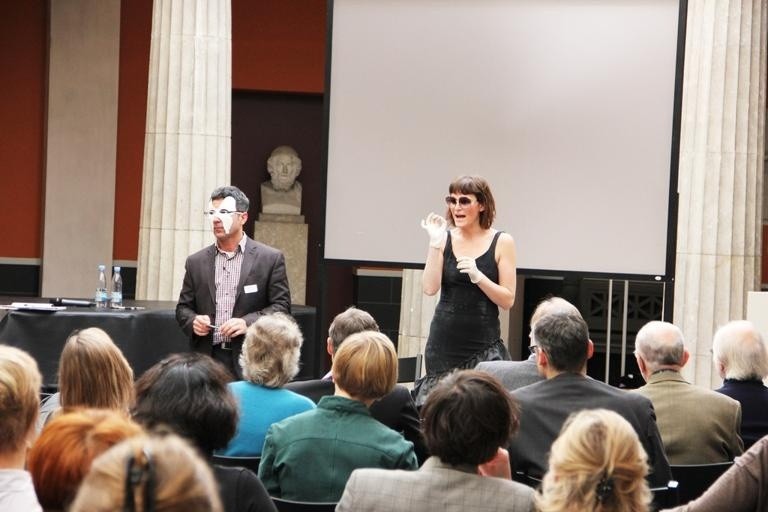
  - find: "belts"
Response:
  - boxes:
[214,342,235,352]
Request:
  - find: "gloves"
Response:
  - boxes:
[455,255,486,285]
[419,211,447,250]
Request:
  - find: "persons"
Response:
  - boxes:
[411,175,518,418]
[177,186,292,380]
[0,298,768,512]
[264,144,302,196]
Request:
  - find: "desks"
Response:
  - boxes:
[0,298,316,392]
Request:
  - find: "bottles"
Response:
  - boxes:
[111,265,123,307]
[95,264,107,309]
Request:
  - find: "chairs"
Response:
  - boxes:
[283,378,335,406]
[211,455,261,474]
[398,354,423,383]
[669,461,734,507]
[515,471,680,512]
[270,496,341,512]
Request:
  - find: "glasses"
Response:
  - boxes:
[444,194,482,210]
